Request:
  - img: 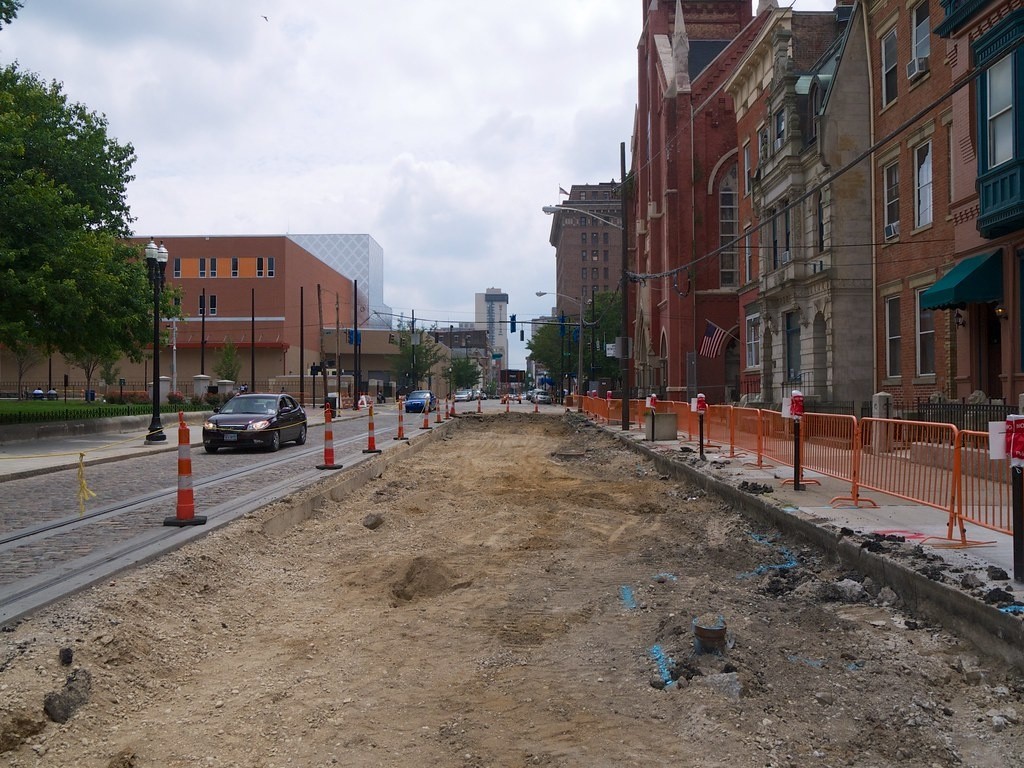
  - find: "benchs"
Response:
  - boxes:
[25,391,58,401]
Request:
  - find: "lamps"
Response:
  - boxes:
[995,300,1008,321]
[954,310,966,328]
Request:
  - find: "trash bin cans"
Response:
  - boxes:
[324,397,337,418]
[84,389,95,401]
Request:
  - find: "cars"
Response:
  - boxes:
[405,390,436,412]
[202,393,308,452]
[454,388,553,404]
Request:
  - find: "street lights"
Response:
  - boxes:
[143,236,169,445]
[535,290,592,395]
[448,368,452,399]
[542,142,630,429]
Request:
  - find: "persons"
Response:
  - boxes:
[33,387,42,393]
[48,388,56,393]
[241,384,248,392]
[377,391,385,400]
[280,387,284,392]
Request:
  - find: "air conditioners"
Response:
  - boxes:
[906,57,926,81]
[774,138,786,152]
[884,224,900,239]
[781,251,792,266]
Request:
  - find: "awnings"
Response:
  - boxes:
[921,248,1003,311]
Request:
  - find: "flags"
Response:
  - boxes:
[699,321,727,359]
